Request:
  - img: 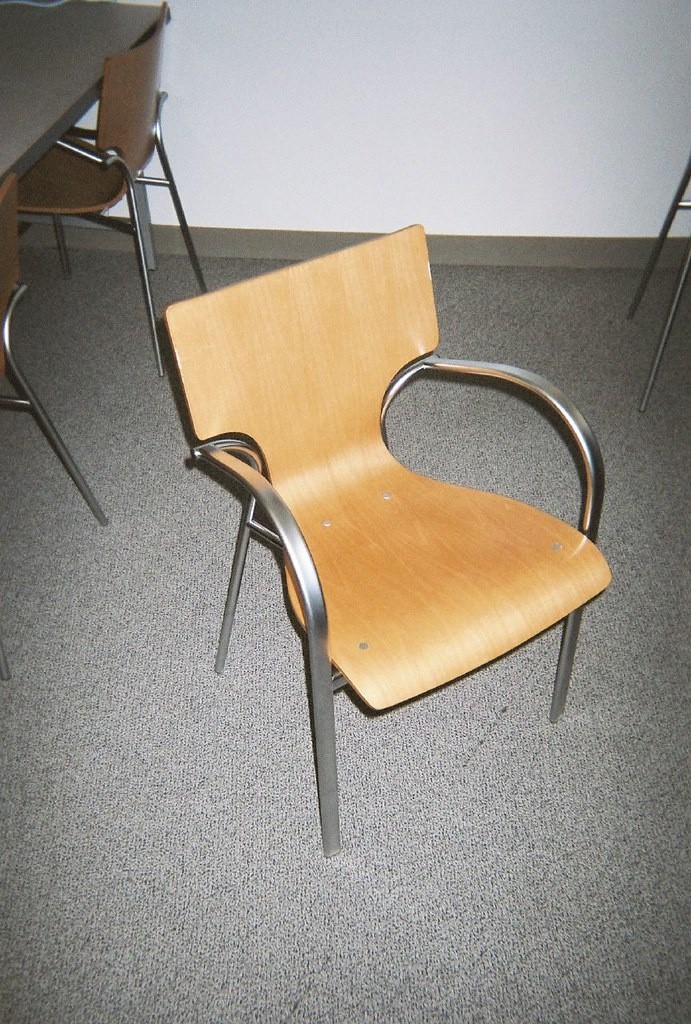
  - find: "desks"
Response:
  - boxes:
[0,0,172,180]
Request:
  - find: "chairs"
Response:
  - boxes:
[0,172,110,682]
[163,221,613,859]
[16,1,209,380]
